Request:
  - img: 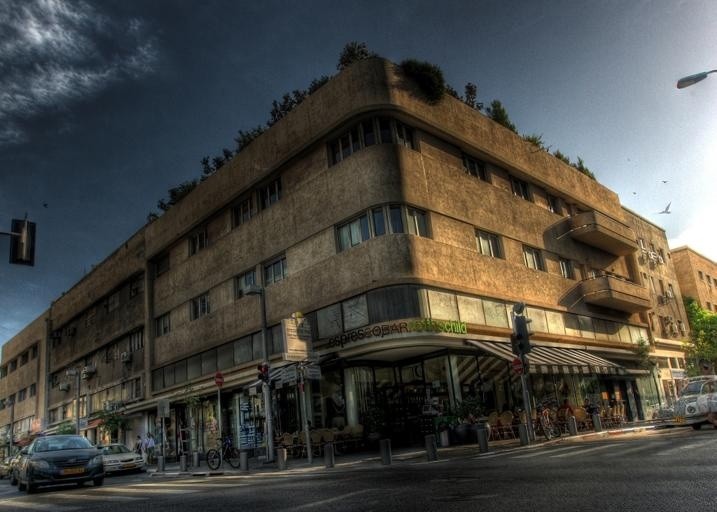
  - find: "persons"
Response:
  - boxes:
[547,403,557,416]
[582,399,595,427]
[67,440,76,448]
[297,419,317,458]
[132,434,143,459]
[39,441,49,451]
[556,399,575,432]
[144,432,155,466]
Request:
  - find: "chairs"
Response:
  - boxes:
[274,428,341,458]
[458,402,625,441]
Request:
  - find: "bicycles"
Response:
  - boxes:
[539,407,562,440]
[206,433,240,470]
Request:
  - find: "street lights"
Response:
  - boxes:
[677,70,717,89]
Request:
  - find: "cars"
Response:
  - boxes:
[0,433,144,494]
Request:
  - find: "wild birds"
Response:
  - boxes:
[662,180,668,184]
[657,200,671,215]
[632,191,637,195]
[42,200,49,208]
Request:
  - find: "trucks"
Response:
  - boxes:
[674,379,717,430]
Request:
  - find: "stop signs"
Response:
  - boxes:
[512,358,524,376]
[214,371,225,387]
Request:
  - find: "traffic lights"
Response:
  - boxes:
[257,365,268,380]
[513,316,536,355]
